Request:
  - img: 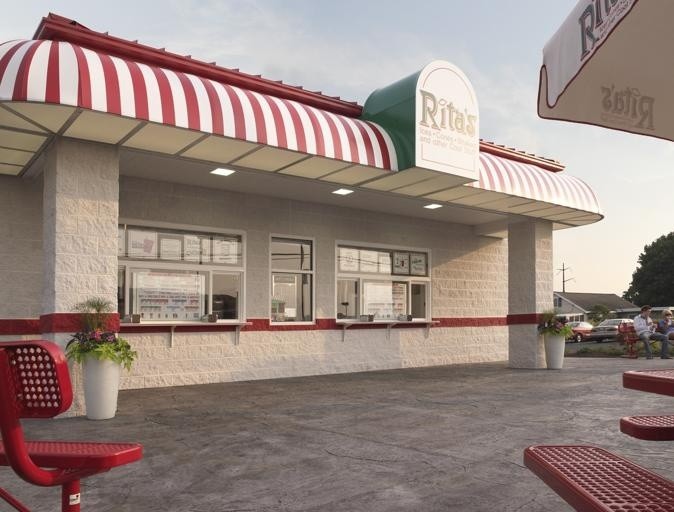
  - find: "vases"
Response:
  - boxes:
[83,352,119,420]
[543,331,566,369]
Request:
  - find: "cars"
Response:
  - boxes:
[563,320,595,344]
[590,317,635,343]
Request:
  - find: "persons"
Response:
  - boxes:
[658,309,674,340]
[633,305,673,359]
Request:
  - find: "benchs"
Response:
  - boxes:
[618,321,673,359]
[521,413,674,509]
[0,336,143,511]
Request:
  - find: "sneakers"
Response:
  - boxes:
[661,355,672,359]
[647,353,653,359]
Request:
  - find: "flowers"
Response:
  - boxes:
[64,297,139,373]
[537,307,574,339]
